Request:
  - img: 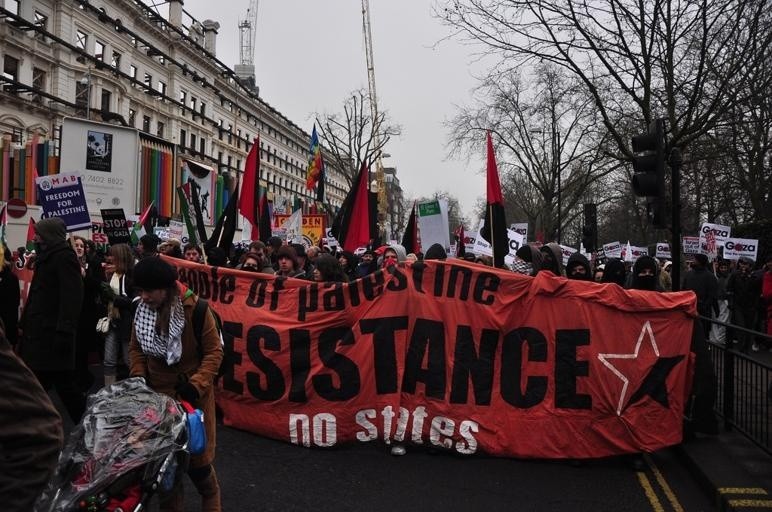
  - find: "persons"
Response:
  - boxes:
[1,216,159,428]
[156,238,721,468]
[126,257,224,511]
[1,335,63,511]
[710,254,771,355]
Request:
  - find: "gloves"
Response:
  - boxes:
[175,382,198,401]
[98,279,116,302]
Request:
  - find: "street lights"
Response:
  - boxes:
[529,127,561,244]
[367,152,391,170]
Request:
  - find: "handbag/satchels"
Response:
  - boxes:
[178,400,207,454]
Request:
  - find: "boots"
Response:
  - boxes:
[193,464,222,511]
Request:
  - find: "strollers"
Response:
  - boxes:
[50,377,189,512]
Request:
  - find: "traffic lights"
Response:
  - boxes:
[632,119,664,199]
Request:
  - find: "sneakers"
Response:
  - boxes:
[390,446,406,455]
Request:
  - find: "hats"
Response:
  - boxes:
[33,217,67,246]
[267,236,282,248]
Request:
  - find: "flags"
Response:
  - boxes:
[278,209,303,244]
[304,122,323,188]
[480,128,510,268]
[317,160,330,204]
[174,130,273,250]
[0,203,8,242]
[329,162,382,254]
[138,200,155,225]
[402,199,450,256]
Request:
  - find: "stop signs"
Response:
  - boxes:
[7,198,27,218]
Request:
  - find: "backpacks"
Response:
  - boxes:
[192,297,232,382]
